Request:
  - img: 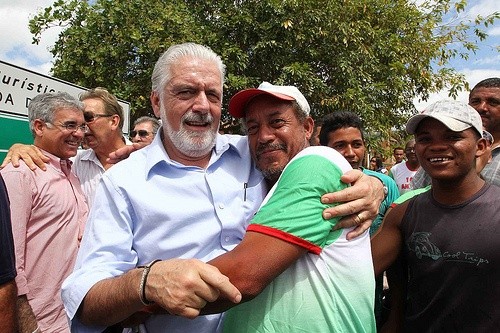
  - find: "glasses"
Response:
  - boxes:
[51,123,89,133]
[83,111,112,122]
[130,130,156,137]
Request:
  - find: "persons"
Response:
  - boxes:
[61,43,388,333]
[106,82,376,333]
[407,78,500,189]
[370,100,500,333]
[0,92,90,333]
[310,111,407,333]
[0,87,128,211]
[130,116,160,144]
[370,139,420,193]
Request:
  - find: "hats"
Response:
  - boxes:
[406,100,483,139]
[229,82,310,119]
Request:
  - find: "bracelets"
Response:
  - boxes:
[138,258,162,305]
[370,174,388,204]
